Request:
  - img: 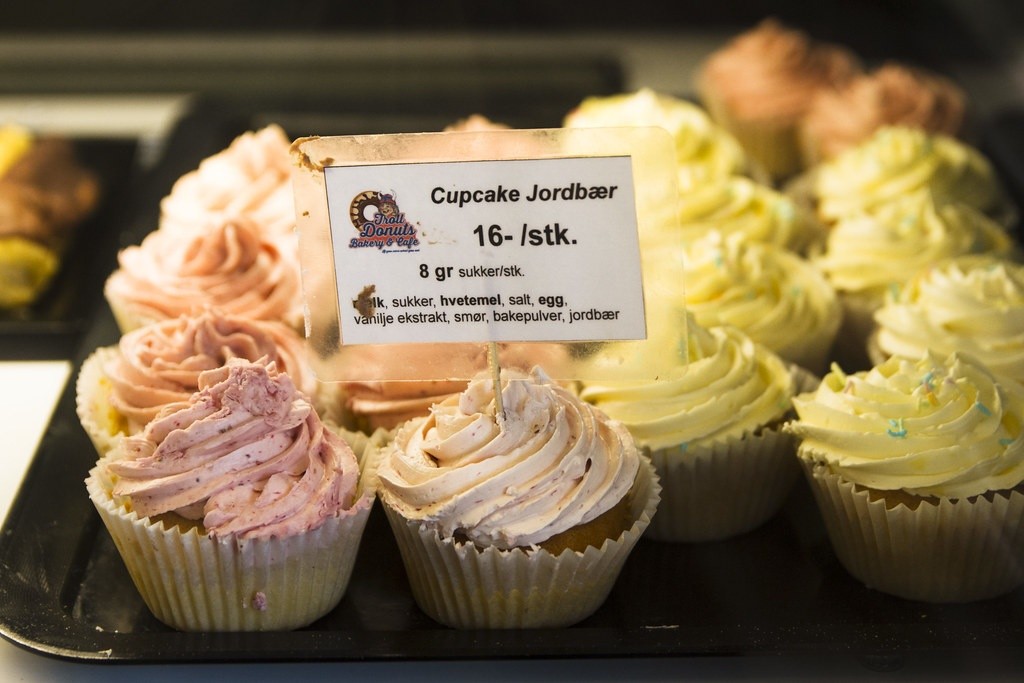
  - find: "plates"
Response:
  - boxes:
[1,135,137,333]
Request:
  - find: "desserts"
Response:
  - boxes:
[72,15,1024,632]
